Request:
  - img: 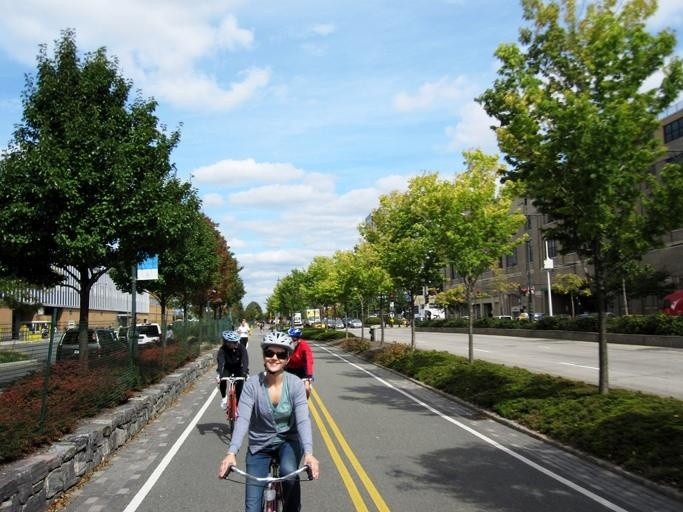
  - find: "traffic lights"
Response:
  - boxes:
[425,295,429,303]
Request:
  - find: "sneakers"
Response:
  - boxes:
[221,395,228,408]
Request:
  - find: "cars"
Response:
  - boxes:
[369,314,389,318]
[304,319,325,329]
[324,317,363,328]
[494,312,545,321]
[56,323,161,363]
[267,319,290,324]
[578,312,615,319]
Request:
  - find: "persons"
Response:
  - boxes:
[215,331,250,413]
[256,319,264,330]
[42,325,48,338]
[240,319,249,326]
[166,324,173,338]
[288,327,315,401]
[238,320,250,349]
[219,331,318,512]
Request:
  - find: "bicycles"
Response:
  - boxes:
[218,376,248,433]
[222,457,313,512]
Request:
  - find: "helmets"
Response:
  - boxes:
[261,332,295,352]
[288,328,302,337]
[223,331,240,342]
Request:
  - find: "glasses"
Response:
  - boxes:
[228,342,238,344]
[264,350,288,359]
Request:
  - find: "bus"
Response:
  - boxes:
[292,313,302,326]
[303,309,320,322]
